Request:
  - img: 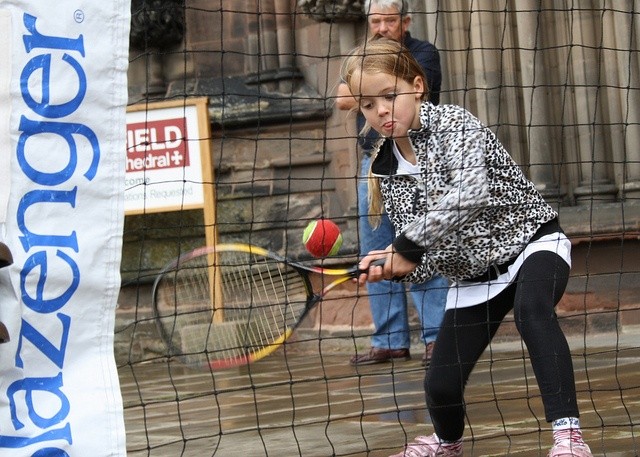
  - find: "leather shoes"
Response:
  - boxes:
[350,348,409,366]
[421,342,435,366]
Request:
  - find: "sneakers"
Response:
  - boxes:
[546,417,592,457]
[389,432,462,457]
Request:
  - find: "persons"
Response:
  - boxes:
[336,0,449,367]
[339,37,593,457]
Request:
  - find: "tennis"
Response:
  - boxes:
[302,218,342,259]
